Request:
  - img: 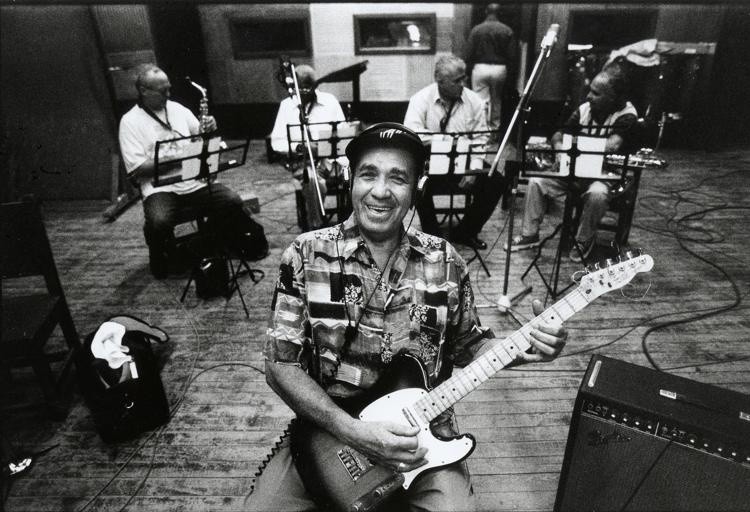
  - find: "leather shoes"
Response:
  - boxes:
[449,228,487,250]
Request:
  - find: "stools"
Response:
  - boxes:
[560,168,644,246]
[502,151,555,213]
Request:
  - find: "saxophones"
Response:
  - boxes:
[525,143,668,170]
[185,76,209,133]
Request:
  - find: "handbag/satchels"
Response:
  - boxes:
[198,242,229,299]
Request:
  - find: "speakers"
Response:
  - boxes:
[552,352,750,512]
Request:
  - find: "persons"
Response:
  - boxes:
[270,66,349,230]
[504,65,638,263]
[463,3,515,148]
[262,122,569,510]
[118,66,245,270]
[404,56,505,249]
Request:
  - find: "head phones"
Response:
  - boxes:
[359,121,429,198]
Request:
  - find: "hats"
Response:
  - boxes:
[345,121,427,166]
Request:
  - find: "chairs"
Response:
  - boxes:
[1,194,83,417]
[139,181,213,265]
[296,176,344,227]
[432,187,471,232]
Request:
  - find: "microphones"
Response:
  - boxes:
[280,55,295,99]
[540,22,563,60]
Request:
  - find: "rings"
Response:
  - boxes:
[397,463,406,472]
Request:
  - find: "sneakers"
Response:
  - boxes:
[570,235,594,262]
[504,232,540,253]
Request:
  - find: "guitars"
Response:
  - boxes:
[291,246,654,510]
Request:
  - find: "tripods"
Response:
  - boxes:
[521,122,593,308]
[180,175,256,320]
[476,161,534,330]
[431,178,492,278]
